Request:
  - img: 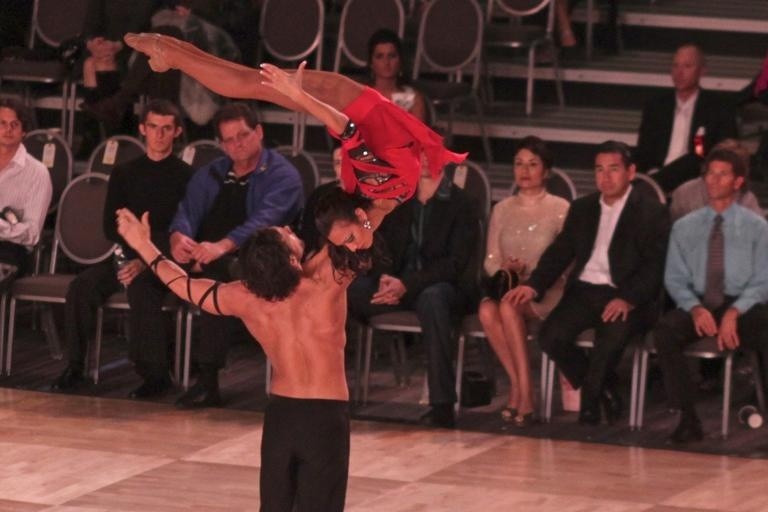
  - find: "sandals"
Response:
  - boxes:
[500,406,516,425]
[515,409,536,427]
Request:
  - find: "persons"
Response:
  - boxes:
[115,208,380,512]
[1,1,768,446]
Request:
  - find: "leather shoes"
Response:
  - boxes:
[51,366,81,390]
[601,390,624,424]
[670,415,703,441]
[129,374,173,399]
[578,401,598,423]
[174,381,221,409]
[421,402,455,427]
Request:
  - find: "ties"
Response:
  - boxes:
[702,215,724,312]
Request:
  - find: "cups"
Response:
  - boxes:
[737,406,762,428]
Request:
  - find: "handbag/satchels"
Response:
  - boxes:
[491,268,518,299]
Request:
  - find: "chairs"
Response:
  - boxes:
[4,172,124,387]
[1,233,48,376]
[636,321,767,439]
[0,0,88,138]
[569,1,625,60]
[482,0,566,115]
[92,283,188,393]
[447,161,491,216]
[178,140,226,167]
[453,324,586,420]
[361,218,501,416]
[85,134,149,176]
[69,78,146,152]
[333,0,407,79]
[276,146,321,194]
[252,0,326,151]
[510,167,578,202]
[347,303,410,408]
[545,331,676,432]
[180,311,272,400]
[17,129,73,270]
[412,1,492,161]
[629,173,668,205]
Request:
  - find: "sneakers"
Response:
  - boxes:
[123,31,168,73]
